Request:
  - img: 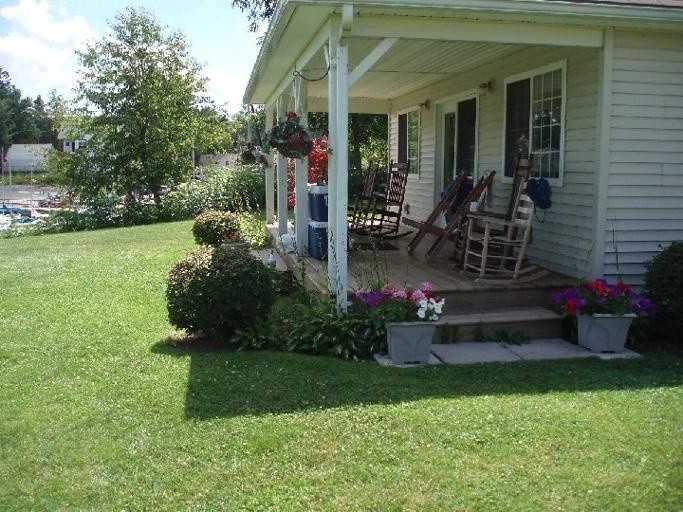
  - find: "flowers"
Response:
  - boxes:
[551,278,658,317]
[356,281,448,322]
[269,112,314,162]
[516,134,529,152]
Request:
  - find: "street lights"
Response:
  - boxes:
[3,158,12,187]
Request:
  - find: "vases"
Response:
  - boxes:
[576,310,636,353]
[385,321,437,365]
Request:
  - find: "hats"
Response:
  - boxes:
[526,177,551,209]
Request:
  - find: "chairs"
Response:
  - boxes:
[348,154,550,285]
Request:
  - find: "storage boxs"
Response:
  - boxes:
[308,218,349,260]
[308,186,328,221]
